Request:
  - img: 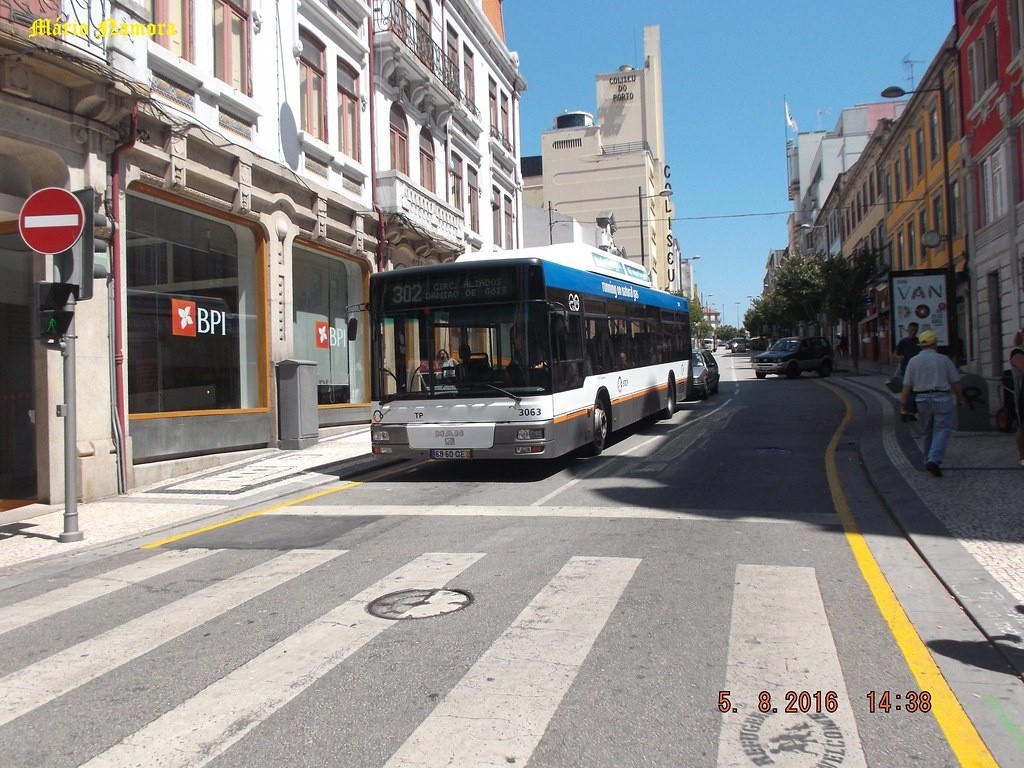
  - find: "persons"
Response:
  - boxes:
[509,336,548,368]
[900,330,967,476]
[1008,327,1024,469]
[435,350,460,378]
[894,322,922,420]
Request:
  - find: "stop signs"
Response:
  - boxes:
[20,188,85,254]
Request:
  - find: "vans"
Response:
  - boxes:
[730,338,746,353]
[703,338,715,353]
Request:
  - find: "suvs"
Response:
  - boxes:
[750,336,834,379]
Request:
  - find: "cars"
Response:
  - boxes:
[691,348,720,399]
[725,341,731,350]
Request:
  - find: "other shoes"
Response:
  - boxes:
[901,412,907,421]
[926,462,943,477]
[908,413,918,421]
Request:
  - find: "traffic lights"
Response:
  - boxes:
[34,280,79,338]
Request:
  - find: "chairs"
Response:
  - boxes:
[462,352,488,381]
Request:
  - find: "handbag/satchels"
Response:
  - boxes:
[885,361,903,393]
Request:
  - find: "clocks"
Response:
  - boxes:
[922,230,941,248]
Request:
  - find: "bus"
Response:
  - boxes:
[345,244,693,462]
[122,288,240,410]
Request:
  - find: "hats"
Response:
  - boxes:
[917,330,937,347]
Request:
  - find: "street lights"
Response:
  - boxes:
[639,189,673,266]
[735,302,740,330]
[879,84,957,363]
[799,222,831,259]
[748,295,752,309]
[701,294,713,305]
[679,255,701,289]
